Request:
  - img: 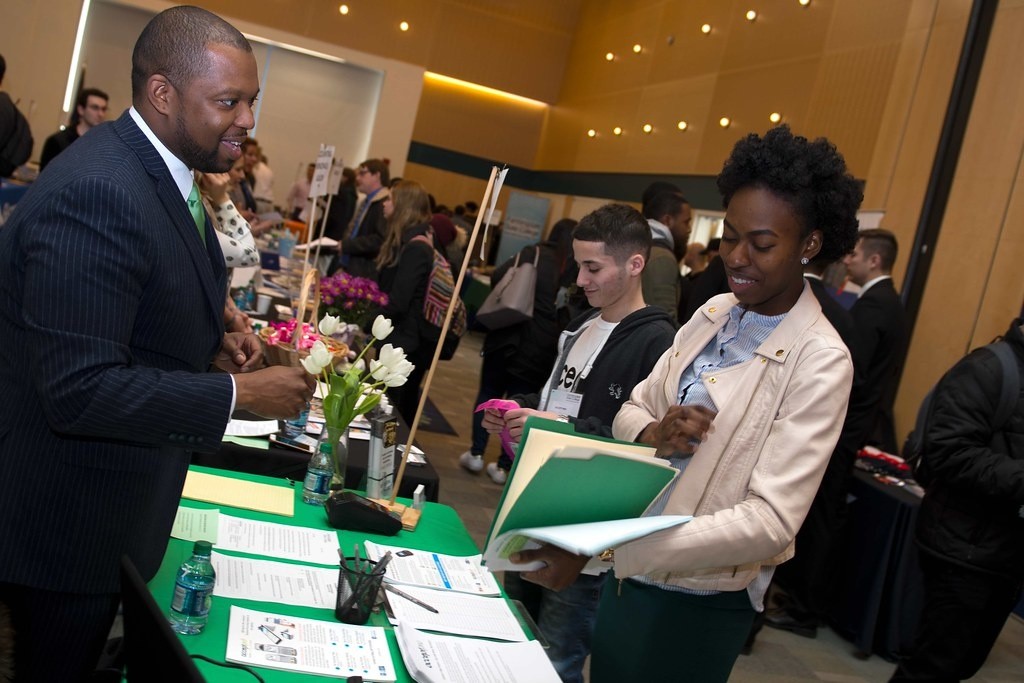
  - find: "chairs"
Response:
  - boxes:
[117,551,264,683]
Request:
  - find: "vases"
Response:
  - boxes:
[312,425,350,496]
[330,330,356,348]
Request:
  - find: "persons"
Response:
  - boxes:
[0,4,317,683]
[885,301,1024,683]
[539,122,868,683]
[481,202,682,683]
[458,179,910,639]
[286,157,487,423]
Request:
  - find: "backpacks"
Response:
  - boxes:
[411,230,466,360]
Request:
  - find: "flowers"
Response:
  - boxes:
[267,270,416,481]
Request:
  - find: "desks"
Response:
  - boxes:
[460,271,492,335]
[145,297,533,683]
[816,468,924,663]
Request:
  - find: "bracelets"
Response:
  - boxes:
[227,315,235,326]
[595,548,614,562]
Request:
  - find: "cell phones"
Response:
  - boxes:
[269,433,315,453]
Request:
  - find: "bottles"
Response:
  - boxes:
[245,280,256,310]
[268,232,278,249]
[234,286,245,311]
[303,444,332,505]
[169,540,216,635]
[284,400,310,439]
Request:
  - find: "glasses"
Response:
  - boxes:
[360,170,369,176]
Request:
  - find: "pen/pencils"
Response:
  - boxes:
[336,548,365,618]
[381,582,439,613]
[338,544,393,621]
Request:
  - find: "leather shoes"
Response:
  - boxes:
[762,609,817,638]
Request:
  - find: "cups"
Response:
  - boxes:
[257,295,272,312]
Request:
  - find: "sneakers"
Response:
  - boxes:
[460,451,484,471]
[488,462,506,484]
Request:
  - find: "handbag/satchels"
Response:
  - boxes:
[476,247,539,329]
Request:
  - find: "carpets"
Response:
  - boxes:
[416,387,459,437]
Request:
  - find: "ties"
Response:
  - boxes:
[187,180,208,249]
[342,199,368,242]
